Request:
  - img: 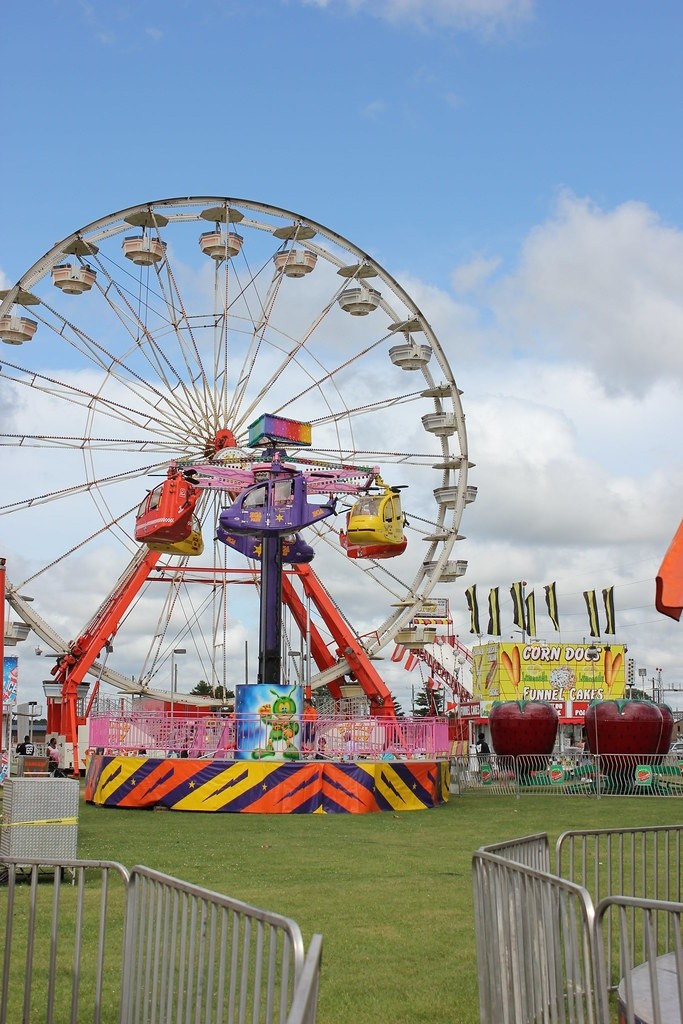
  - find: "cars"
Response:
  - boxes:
[669,743,683,759]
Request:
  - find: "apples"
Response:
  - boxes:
[489,698,557,774]
[585,700,675,778]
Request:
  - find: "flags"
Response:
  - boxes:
[487,587,502,636]
[583,590,600,637]
[465,584,481,635]
[542,582,560,633]
[602,587,615,634]
[510,582,536,637]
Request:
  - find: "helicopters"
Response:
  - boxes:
[338,485,409,544]
[134,469,203,543]
[334,503,410,559]
[218,471,338,539]
[148,512,205,556]
[213,506,315,563]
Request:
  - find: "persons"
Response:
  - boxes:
[313,737,331,760]
[476,733,491,783]
[16,735,34,756]
[96,745,188,758]
[46,738,60,772]
[301,699,318,750]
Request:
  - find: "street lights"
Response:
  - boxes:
[170,649,187,734]
[515,630,525,644]
[656,668,662,703]
[28,701,37,743]
[288,651,302,686]
[639,669,646,700]
